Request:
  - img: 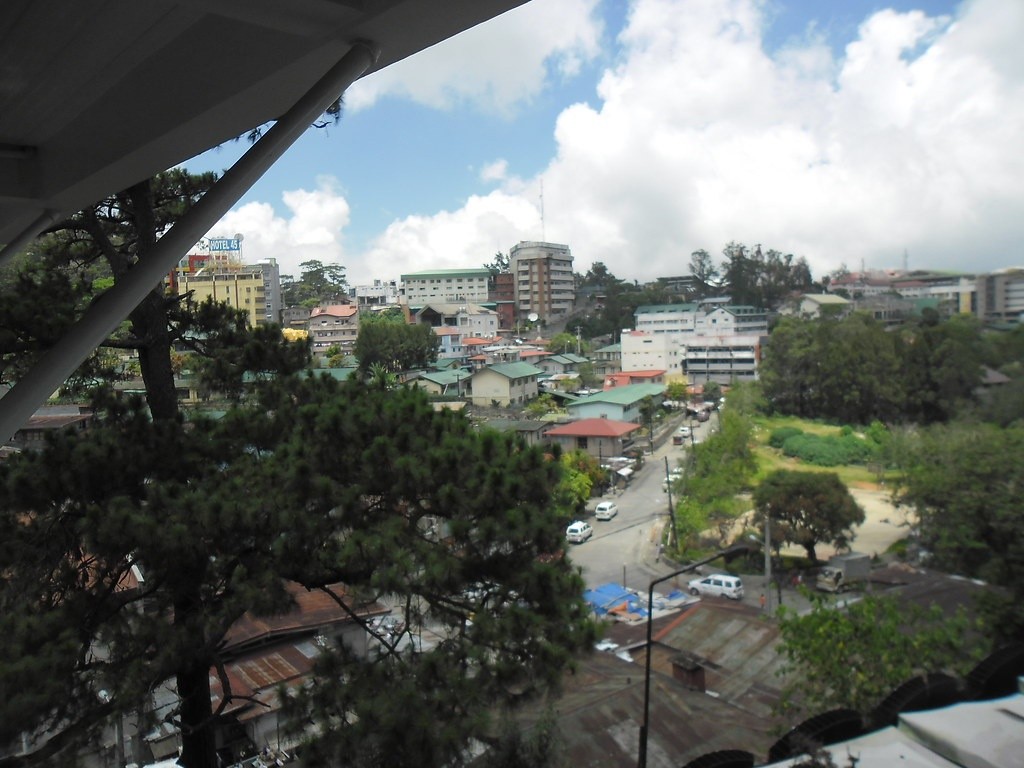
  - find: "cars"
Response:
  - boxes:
[662,466,685,493]
[698,411,712,422]
[680,426,692,438]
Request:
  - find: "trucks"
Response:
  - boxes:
[816,552,870,594]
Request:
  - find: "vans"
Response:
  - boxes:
[689,573,744,600]
[594,501,618,522]
[566,520,593,544]
